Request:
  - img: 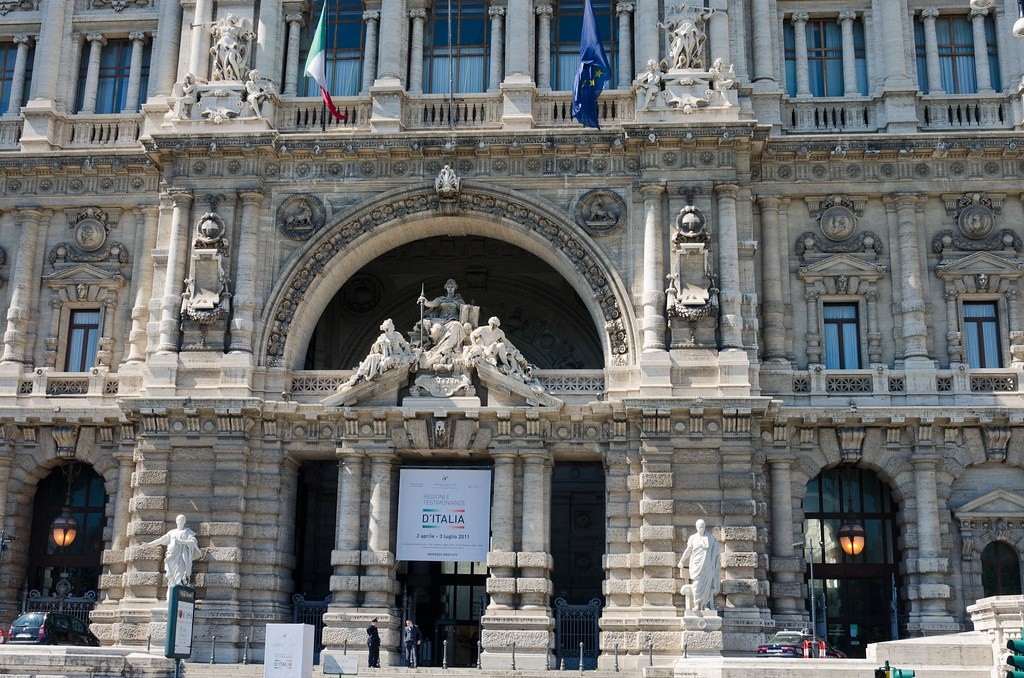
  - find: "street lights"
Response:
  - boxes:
[791,539,824,658]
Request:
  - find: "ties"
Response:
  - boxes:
[410,626,412,630]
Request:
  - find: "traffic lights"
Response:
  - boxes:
[875,668,886,678]
[893,668,915,678]
[1006,628,1024,678]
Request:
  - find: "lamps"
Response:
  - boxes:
[840,474,865,560]
[51,463,82,554]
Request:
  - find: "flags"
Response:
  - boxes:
[573,0,618,129]
[304,0,350,120]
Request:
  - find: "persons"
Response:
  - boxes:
[350,319,407,385]
[403,620,420,668]
[203,14,256,83]
[173,73,199,119]
[416,280,472,345]
[656,0,715,68]
[367,618,382,667]
[709,57,736,107]
[630,59,661,108]
[139,515,202,599]
[246,69,270,118]
[677,518,722,609]
[471,315,533,382]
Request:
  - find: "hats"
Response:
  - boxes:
[372,618,377,622]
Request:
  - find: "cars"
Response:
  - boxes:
[756,630,848,658]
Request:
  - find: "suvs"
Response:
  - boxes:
[5,611,101,648]
